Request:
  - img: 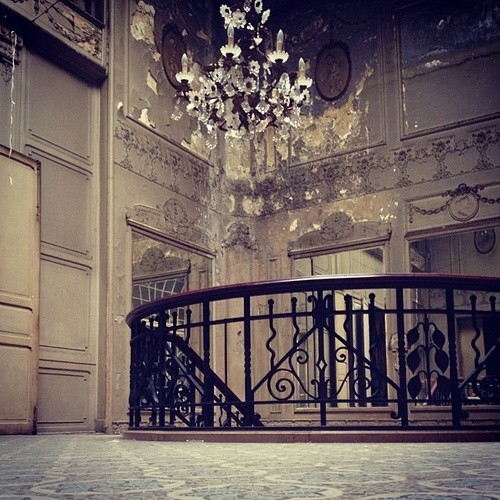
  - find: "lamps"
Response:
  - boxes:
[172,0,314,143]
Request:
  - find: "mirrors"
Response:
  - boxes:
[132,230,190,413]
[290,245,391,409]
[403,228,500,409]
